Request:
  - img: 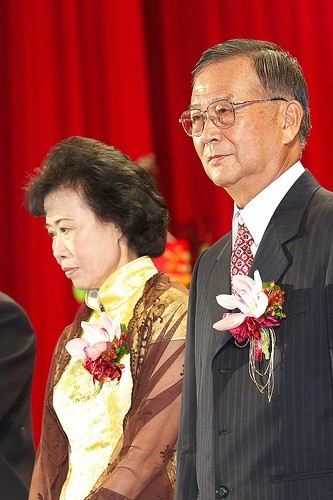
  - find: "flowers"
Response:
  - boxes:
[65,311,129,393]
[213,270,287,402]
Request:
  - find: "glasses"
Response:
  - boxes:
[179,97,288,136]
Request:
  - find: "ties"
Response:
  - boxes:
[230,224,254,295]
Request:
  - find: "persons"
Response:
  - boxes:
[178,38,332,499]
[22,135,188,500]
[0,291,36,499]
